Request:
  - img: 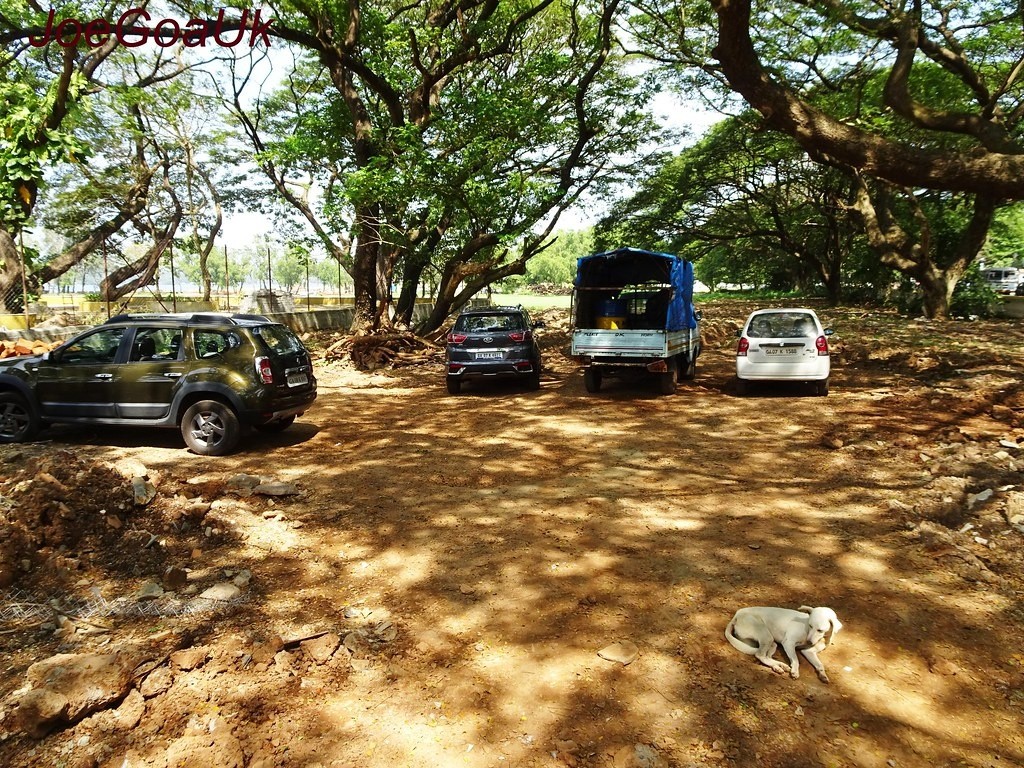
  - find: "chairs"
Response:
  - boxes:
[756,318,809,337]
[164,335,181,360]
[139,338,156,361]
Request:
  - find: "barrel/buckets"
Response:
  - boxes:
[594,299,627,329]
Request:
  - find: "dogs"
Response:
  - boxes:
[724,604,842,684]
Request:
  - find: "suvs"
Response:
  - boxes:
[0,312,317,456]
[445,303,545,394]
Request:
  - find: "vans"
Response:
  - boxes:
[982,267,1019,295]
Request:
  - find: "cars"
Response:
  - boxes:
[736,308,834,396]
[1016,276,1024,297]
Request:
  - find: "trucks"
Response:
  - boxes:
[570,247,703,393]
